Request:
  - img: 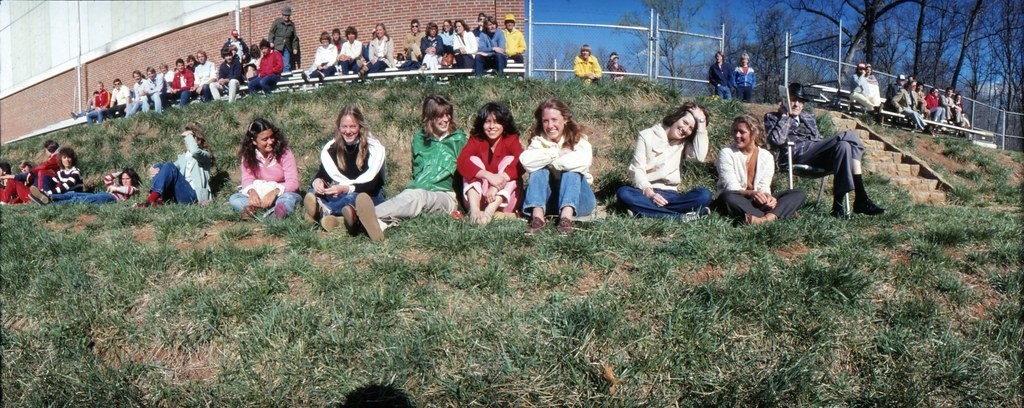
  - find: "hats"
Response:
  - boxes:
[505,14,515,21]
[251,45,259,52]
[789,63,916,101]
[260,39,270,49]
[230,29,240,36]
[282,8,291,15]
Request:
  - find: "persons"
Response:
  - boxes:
[607,52,628,83]
[0,139,81,207]
[300,23,395,85]
[574,41,602,86]
[401,12,538,76]
[302,95,595,244]
[230,117,303,225]
[25,168,144,211]
[712,113,806,226]
[69,25,285,127]
[707,50,759,103]
[849,63,979,136]
[268,8,303,74]
[763,81,884,216]
[129,126,219,209]
[616,103,716,223]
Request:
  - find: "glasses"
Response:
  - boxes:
[114,83,120,85]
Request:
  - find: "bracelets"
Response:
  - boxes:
[248,189,254,195]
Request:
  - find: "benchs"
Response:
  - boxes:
[810,83,967,134]
[234,57,527,93]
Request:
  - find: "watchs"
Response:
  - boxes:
[697,118,706,123]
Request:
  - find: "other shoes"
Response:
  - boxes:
[302,73,310,83]
[685,207,711,222]
[342,204,365,235]
[269,202,286,221]
[321,215,345,233]
[627,209,640,219]
[132,202,148,208]
[317,71,325,78]
[745,213,752,225]
[356,193,385,241]
[532,216,546,229]
[71,111,79,120]
[831,208,848,220]
[853,199,886,215]
[28,186,50,207]
[558,216,572,235]
[241,206,256,220]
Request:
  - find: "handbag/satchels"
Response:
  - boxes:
[442,53,453,66]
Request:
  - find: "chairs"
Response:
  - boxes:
[762,122,853,218]
